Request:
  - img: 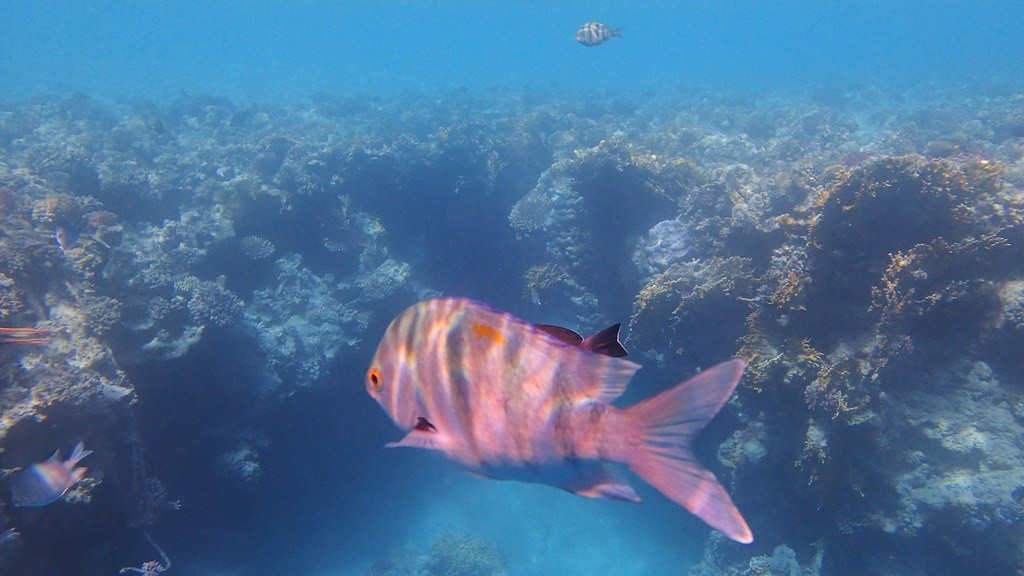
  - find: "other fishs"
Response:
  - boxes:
[574,21,621,46]
[10,441,93,508]
[364,297,755,545]
